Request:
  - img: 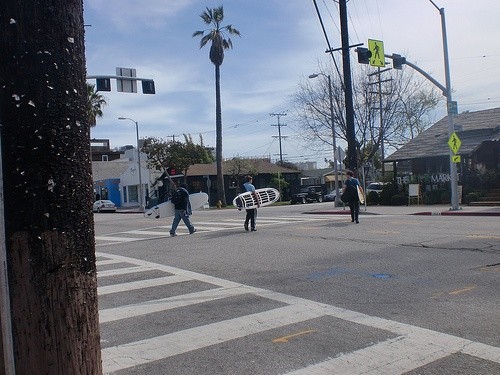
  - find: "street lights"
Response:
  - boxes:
[308,72,343,202]
[118,117,145,212]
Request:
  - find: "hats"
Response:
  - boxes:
[180,183,188,189]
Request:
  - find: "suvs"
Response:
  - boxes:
[289,187,323,205]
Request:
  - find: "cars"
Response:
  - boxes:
[323,187,343,202]
[93,199,117,213]
[365,181,391,197]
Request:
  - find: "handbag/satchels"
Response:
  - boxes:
[340,180,357,203]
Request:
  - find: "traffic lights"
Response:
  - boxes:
[357,46,372,64]
[393,53,406,69]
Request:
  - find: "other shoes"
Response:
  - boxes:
[191,229,197,234]
[251,227,257,232]
[356,220,359,224]
[170,232,177,237]
[244,223,249,231]
[350,220,354,223]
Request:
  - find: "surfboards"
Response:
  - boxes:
[357,185,365,204]
[144,192,209,219]
[232,188,280,210]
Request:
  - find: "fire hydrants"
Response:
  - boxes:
[216,199,223,209]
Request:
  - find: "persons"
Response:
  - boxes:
[343,171,364,224]
[240,176,260,231]
[170,183,196,236]
